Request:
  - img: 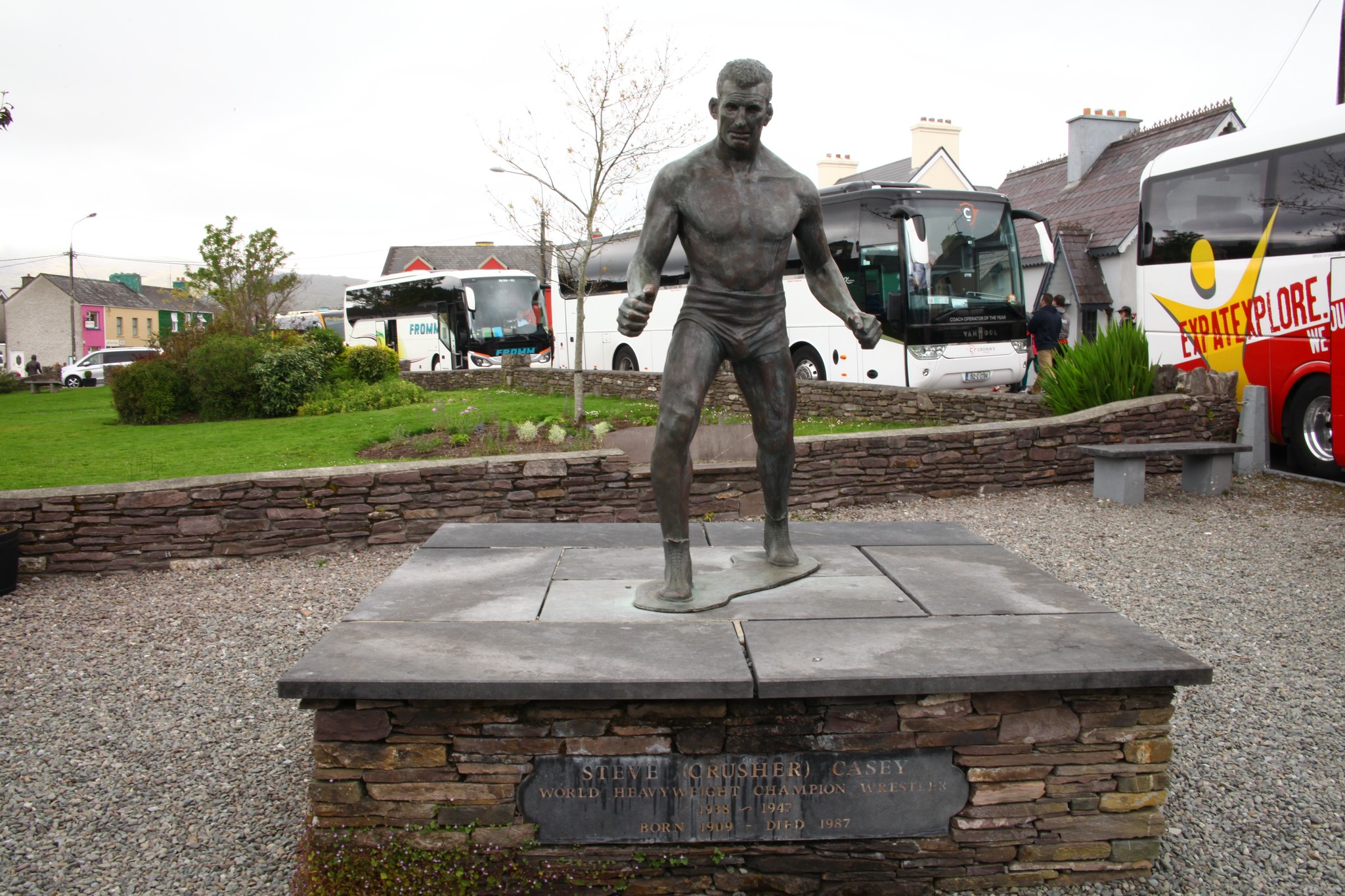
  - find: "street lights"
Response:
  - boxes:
[68,212,98,364]
[490,166,547,284]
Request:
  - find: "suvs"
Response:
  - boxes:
[60,346,166,389]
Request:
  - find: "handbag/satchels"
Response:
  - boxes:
[1027,345,1035,360]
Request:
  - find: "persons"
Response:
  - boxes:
[511,310,528,329]
[1052,294,1071,370]
[1027,294,1059,394]
[991,293,1048,393]
[1114,305,1136,332]
[548,330,554,367]
[25,355,43,389]
[617,59,884,599]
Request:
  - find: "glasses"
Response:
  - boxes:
[932,257,938,262]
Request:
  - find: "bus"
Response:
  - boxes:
[1134,100,1345,489]
[540,177,1056,391]
[247,309,346,348]
[342,269,552,372]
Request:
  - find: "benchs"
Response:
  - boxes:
[1075,440,1254,506]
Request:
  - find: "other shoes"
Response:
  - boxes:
[991,387,1001,393]
[1018,387,1025,392]
[964,388,975,391]
[1025,388,1033,395]
[1005,388,1018,394]
[1040,388,1045,393]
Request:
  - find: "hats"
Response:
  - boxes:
[1115,305,1131,312]
